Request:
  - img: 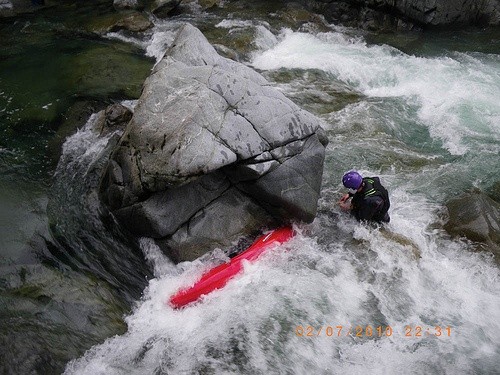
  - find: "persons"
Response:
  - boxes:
[339,171,390,223]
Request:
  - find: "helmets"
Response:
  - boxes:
[343,171,362,190]
